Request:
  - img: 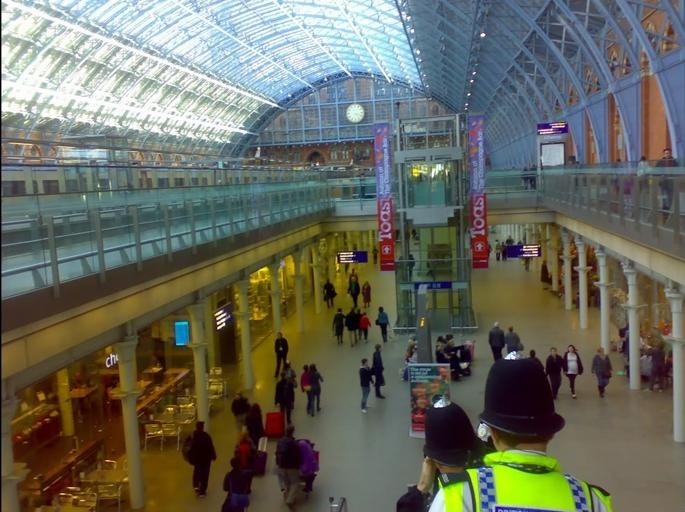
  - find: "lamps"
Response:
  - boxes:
[464,24,487,110]
[399,0,432,100]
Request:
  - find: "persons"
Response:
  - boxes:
[521,148,679,225]
[372,246,380,264]
[529,319,670,398]
[180,393,307,511]
[409,255,438,281]
[489,323,524,361]
[358,173,365,198]
[540,260,595,309]
[321,269,391,347]
[396,351,612,511]
[436,333,472,381]
[359,344,387,413]
[273,333,324,426]
[400,340,418,381]
[487,235,530,272]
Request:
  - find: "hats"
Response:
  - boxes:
[422,396,479,457]
[479,349,566,438]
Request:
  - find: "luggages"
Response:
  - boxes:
[263,404,285,439]
[252,436,268,476]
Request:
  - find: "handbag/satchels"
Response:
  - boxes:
[228,492,249,508]
[181,443,194,463]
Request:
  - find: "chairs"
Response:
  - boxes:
[141,368,227,451]
[44,456,128,512]
[247,286,285,307]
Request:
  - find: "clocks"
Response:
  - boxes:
[346,104,365,123]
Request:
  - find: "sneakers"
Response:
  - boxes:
[307,394,386,417]
[191,486,208,498]
